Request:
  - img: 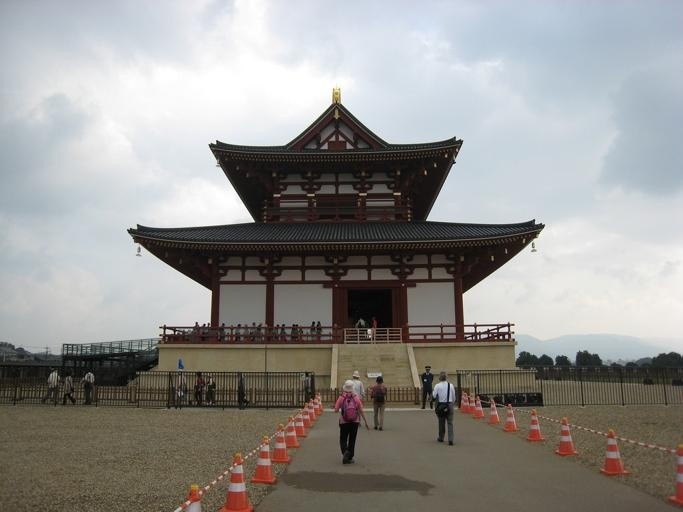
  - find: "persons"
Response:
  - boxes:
[194,375,205,407]
[206,377,216,406]
[371,316,378,328]
[193,322,322,341]
[431,371,457,445]
[420,365,434,409]
[365,317,370,327]
[299,373,311,401]
[334,379,371,463]
[370,376,387,431]
[80,369,95,405]
[41,369,61,404]
[236,373,250,407]
[356,316,365,328]
[174,371,187,403]
[60,372,77,404]
[352,369,366,427]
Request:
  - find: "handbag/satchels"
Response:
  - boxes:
[435,403,450,417]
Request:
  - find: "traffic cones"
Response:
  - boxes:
[554,417,579,456]
[285,393,323,449]
[271,424,291,463]
[525,408,546,442]
[668,445,682,506]
[501,403,522,433]
[599,429,630,476]
[459,391,500,425]
[186,484,203,512]
[250,435,276,485]
[217,451,254,512]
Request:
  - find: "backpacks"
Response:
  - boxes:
[375,390,385,404]
[339,394,361,422]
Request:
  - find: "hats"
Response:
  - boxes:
[424,365,431,369]
[342,380,355,393]
[352,371,360,378]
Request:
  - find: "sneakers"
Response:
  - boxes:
[374,426,383,431]
[342,455,355,464]
[437,438,454,446]
